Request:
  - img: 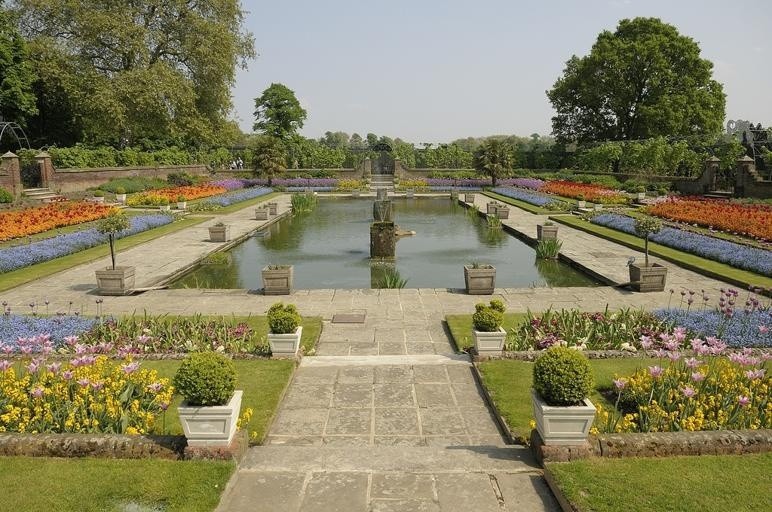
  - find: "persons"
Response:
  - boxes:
[229,160,237,169]
[237,156,243,170]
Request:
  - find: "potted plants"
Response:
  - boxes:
[406,186,415,198]
[268,201,278,215]
[168,351,245,446]
[495,204,509,219]
[470,300,508,356]
[160,194,188,212]
[530,345,597,444]
[537,221,558,242]
[636,186,668,203]
[208,221,230,242]
[450,187,459,199]
[351,186,360,197]
[628,213,668,291]
[265,300,303,356]
[97,207,136,294]
[93,185,126,203]
[576,192,603,213]
[487,201,499,215]
[261,262,294,295]
[464,258,496,295]
[255,205,270,220]
[465,192,475,203]
